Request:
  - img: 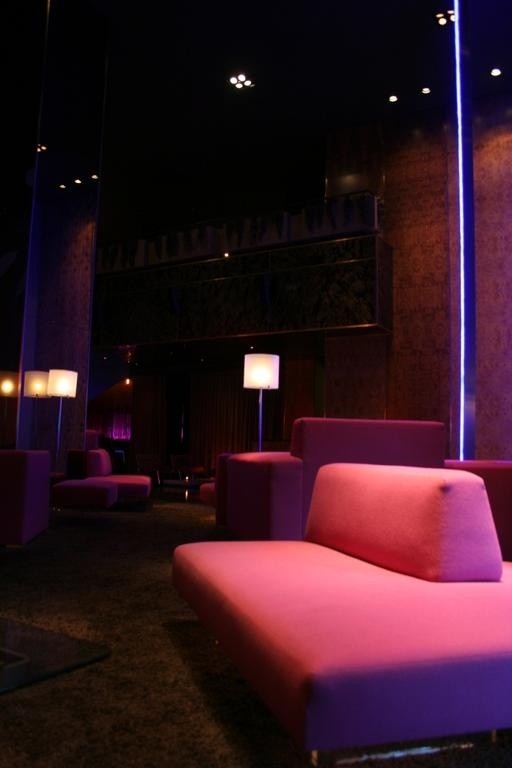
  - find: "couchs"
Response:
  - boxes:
[170,417,511,765]
[51,447,152,506]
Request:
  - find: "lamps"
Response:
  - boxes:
[23,370,50,451]
[45,368,79,471]
[240,354,281,451]
